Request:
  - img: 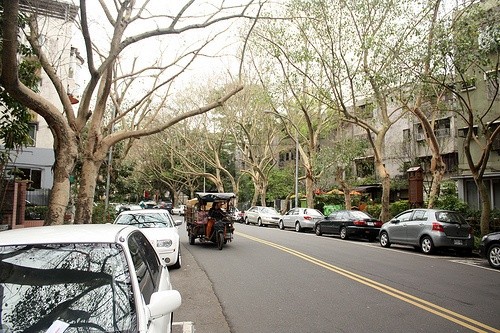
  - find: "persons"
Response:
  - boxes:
[205,202,228,239]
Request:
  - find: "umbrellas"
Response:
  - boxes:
[290,190,362,198]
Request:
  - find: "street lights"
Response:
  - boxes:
[105,74,161,213]
[263,109,300,208]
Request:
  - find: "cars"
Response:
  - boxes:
[105,209,184,269]
[170,204,186,217]
[276,208,326,231]
[232,205,283,226]
[480,231,500,270]
[0,223,182,333]
[378,208,475,258]
[312,210,382,240]
[108,200,172,214]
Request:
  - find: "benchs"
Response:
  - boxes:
[196,221,208,225]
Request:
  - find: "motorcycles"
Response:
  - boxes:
[184,191,238,250]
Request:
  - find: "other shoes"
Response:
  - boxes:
[205,237,209,240]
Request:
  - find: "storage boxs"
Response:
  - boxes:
[186,197,227,211]
